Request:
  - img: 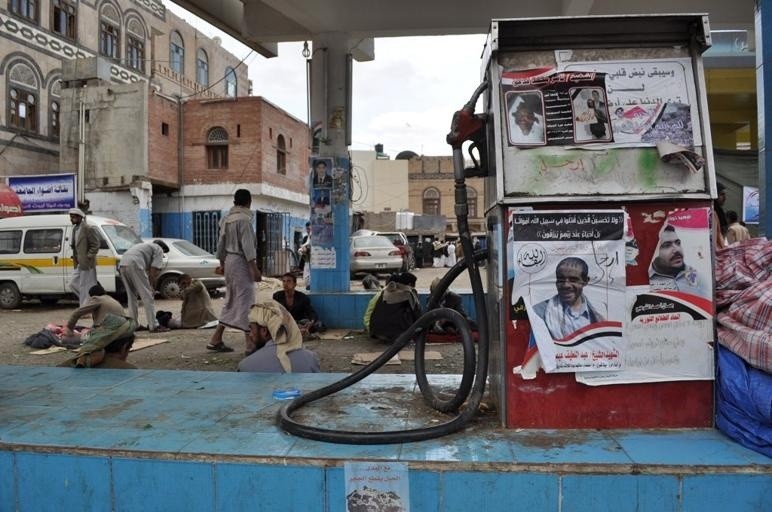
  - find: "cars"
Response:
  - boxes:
[348,232,416,279]
[142,238,225,300]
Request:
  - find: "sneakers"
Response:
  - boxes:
[206,341,235,353]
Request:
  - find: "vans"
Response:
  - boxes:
[0,215,143,309]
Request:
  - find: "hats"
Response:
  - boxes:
[69,207,86,218]
[153,239,170,253]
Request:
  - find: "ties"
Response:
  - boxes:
[320,178,323,183]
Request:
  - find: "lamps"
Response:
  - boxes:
[301,41,310,57]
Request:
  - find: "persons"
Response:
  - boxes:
[206,188,262,356]
[66,285,125,336]
[531,257,605,340]
[592,90,607,123]
[726,210,751,244]
[511,107,544,143]
[273,221,482,347]
[313,161,333,187]
[614,108,631,131]
[235,299,320,373]
[167,274,218,329]
[585,98,607,140]
[714,182,728,249]
[316,189,330,207]
[67,208,100,318]
[649,225,704,294]
[119,239,171,333]
[92,332,138,369]
